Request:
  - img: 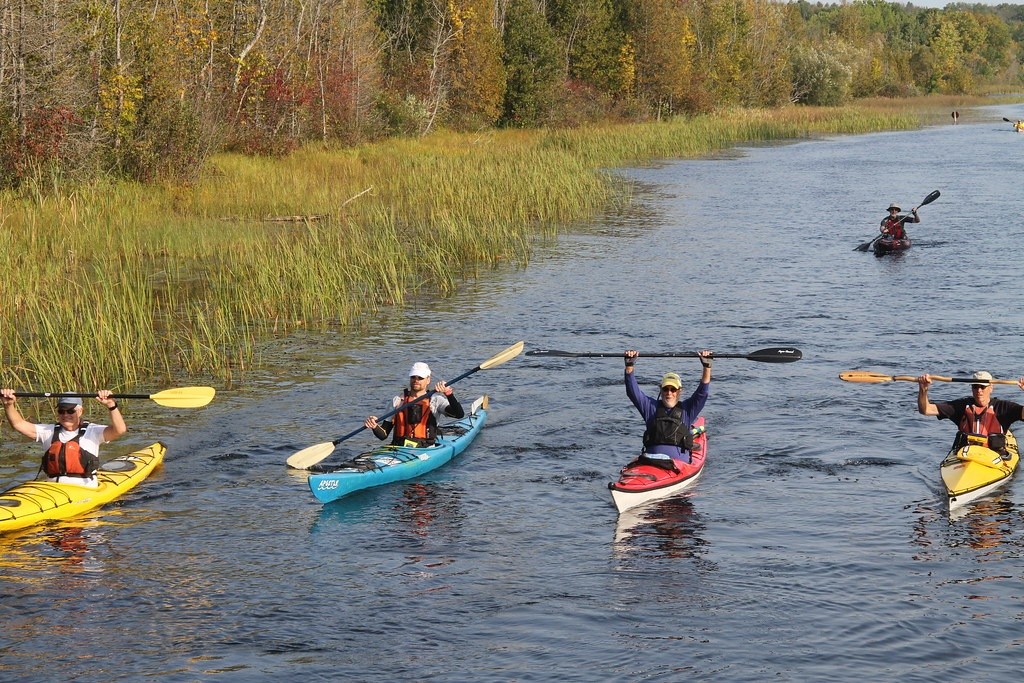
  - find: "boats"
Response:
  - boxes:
[0,440,167,534]
[308,395,488,505]
[873,234,911,249]
[608,415,707,513]
[939,428,1019,511]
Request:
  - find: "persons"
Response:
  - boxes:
[1013,119,1024,132]
[624,350,713,464]
[917,370,1024,456]
[0,389,127,487]
[364,362,464,448]
[880,203,920,240]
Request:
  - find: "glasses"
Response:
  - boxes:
[411,376,424,380]
[662,386,678,393]
[58,409,80,414]
[972,384,990,390]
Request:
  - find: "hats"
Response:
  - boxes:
[886,202,901,212]
[409,362,431,378]
[662,372,682,390]
[969,371,992,386]
[54,392,82,409]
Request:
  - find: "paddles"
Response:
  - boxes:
[838,370,1023,391]
[286,340,525,469]
[525,348,803,363]
[1003,117,1016,125]
[853,190,941,251]
[1,386,216,408]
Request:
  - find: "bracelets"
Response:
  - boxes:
[108,402,118,411]
